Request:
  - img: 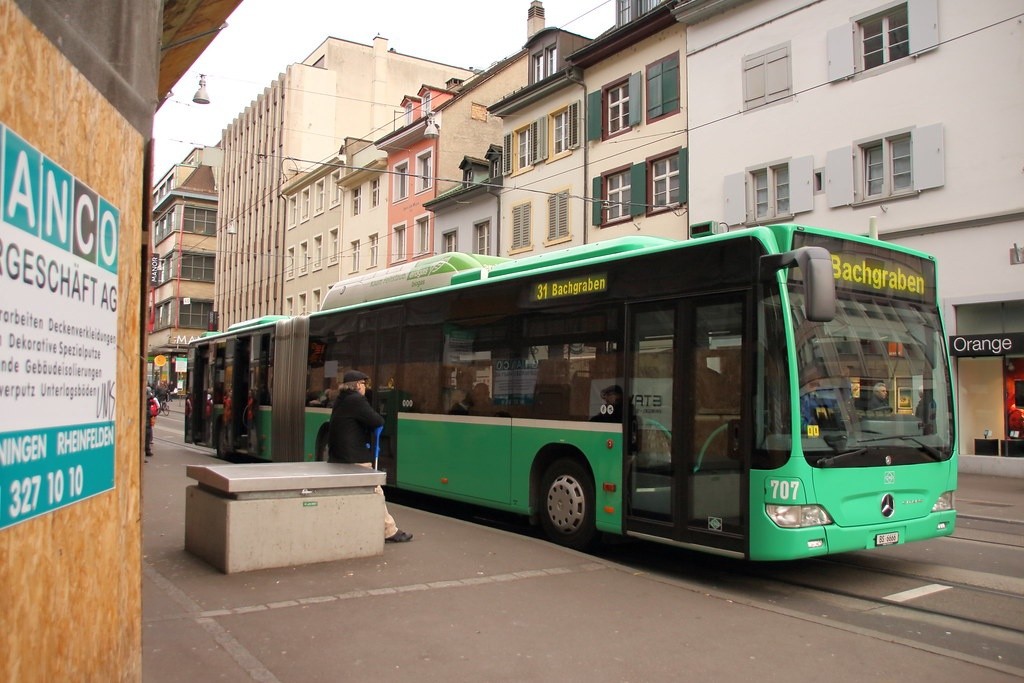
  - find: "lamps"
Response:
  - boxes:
[192,73,210,105]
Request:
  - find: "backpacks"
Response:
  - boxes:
[150,399,158,417]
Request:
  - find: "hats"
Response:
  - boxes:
[343,370,368,383]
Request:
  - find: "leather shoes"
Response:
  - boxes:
[385,529,413,542]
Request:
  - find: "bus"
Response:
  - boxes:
[183,219,961,563]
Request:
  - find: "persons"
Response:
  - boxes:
[223,387,232,443]
[146,387,160,457]
[308,377,334,408]
[796,367,822,435]
[327,369,412,542]
[206,394,212,439]
[865,383,892,414]
[185,393,192,437]
[247,389,255,423]
[591,385,641,473]
[169,381,175,391]
[915,385,938,434]
[448,372,497,415]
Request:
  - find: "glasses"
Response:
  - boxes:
[358,382,367,384]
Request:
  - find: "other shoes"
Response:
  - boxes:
[146,452,153,455]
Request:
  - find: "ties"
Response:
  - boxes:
[813,394,819,404]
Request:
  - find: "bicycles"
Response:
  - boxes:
[157,401,170,416]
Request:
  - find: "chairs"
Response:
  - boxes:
[571,370,591,389]
